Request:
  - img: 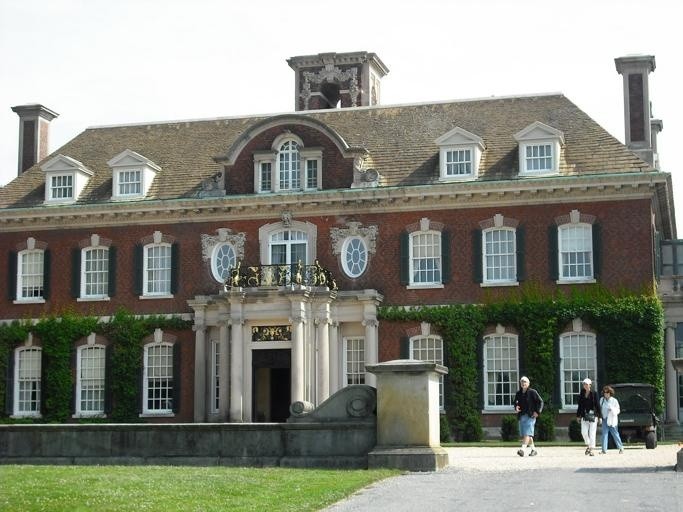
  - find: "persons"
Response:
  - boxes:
[514,375,544,457]
[599,386,624,455]
[576,377,602,456]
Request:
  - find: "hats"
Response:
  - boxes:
[582,378,592,385]
[520,376,529,383]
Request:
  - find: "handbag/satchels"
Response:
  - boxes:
[584,410,595,422]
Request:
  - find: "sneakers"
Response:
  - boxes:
[584,448,625,456]
[517,449,537,457]
